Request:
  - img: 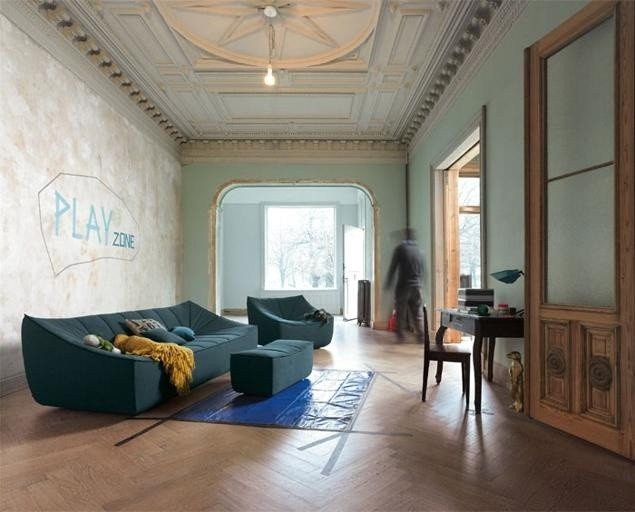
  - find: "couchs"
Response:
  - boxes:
[21,298,259,416]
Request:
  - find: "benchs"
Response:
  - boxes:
[230,339,314,397]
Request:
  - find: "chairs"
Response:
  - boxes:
[421,303,472,410]
[246,294,334,349]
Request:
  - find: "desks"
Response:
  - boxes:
[433,307,525,415]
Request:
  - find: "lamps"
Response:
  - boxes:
[488,269,525,285]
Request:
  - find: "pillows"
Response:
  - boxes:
[122,317,195,346]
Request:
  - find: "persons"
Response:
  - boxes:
[382,228,427,344]
